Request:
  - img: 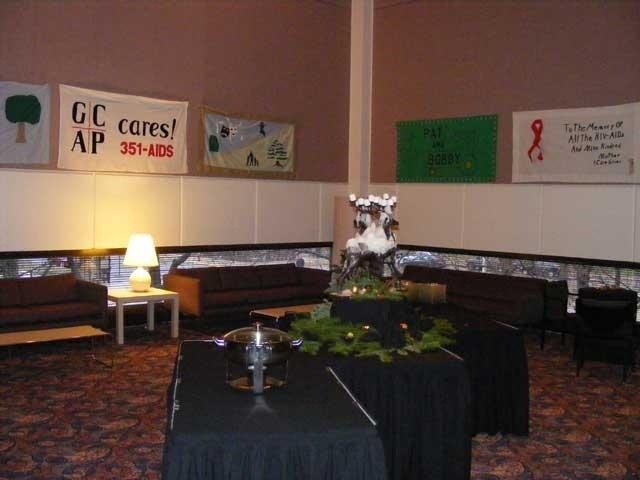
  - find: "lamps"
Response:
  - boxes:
[122,235,160,291]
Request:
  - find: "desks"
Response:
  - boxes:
[1,323,114,370]
[250,303,330,327]
[105,288,180,346]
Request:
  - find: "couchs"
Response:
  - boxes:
[0,275,109,328]
[401,264,569,351]
[164,263,330,320]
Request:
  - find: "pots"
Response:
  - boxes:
[210,321,305,394]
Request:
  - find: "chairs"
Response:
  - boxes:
[574,288,638,381]
